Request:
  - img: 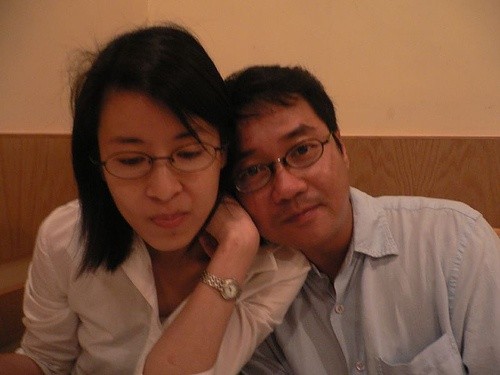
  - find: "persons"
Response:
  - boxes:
[221,65,500,375]
[0,25,311,375]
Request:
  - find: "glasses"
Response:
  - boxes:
[99,142,223,179]
[232,129,333,194]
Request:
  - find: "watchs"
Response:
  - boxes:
[198,270,243,302]
